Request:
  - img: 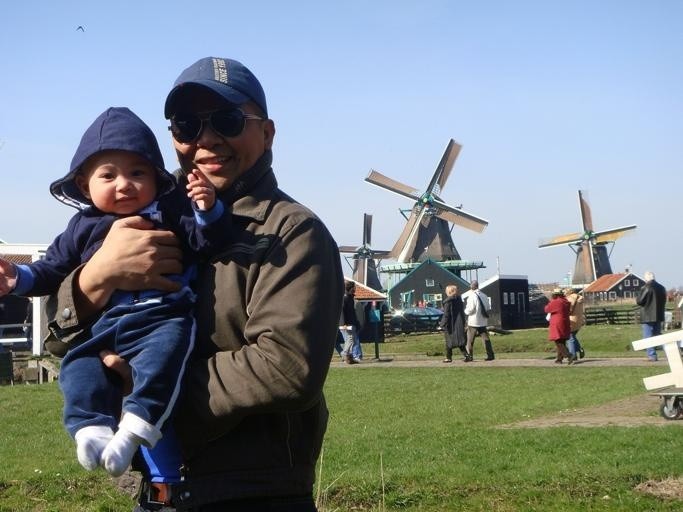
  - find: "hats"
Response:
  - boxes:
[164,58,266,115]
[551,288,563,295]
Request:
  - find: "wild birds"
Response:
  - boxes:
[76,26,84,32]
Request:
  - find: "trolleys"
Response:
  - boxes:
[644,389,683,420]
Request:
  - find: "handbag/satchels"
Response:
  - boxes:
[480,303,489,318]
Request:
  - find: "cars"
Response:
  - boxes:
[390,308,444,334]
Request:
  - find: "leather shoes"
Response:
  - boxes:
[555,360,562,364]
[568,355,576,364]
[442,355,473,362]
[343,355,352,365]
[580,348,584,358]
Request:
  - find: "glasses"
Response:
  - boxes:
[167,106,263,144]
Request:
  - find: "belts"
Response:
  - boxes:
[137,480,177,506]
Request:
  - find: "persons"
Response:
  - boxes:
[42,56,343,512]
[462,281,495,361]
[0,108,225,479]
[335,281,363,364]
[24,301,52,382]
[636,271,666,360]
[543,289,575,364]
[437,286,472,363]
[562,287,584,359]
